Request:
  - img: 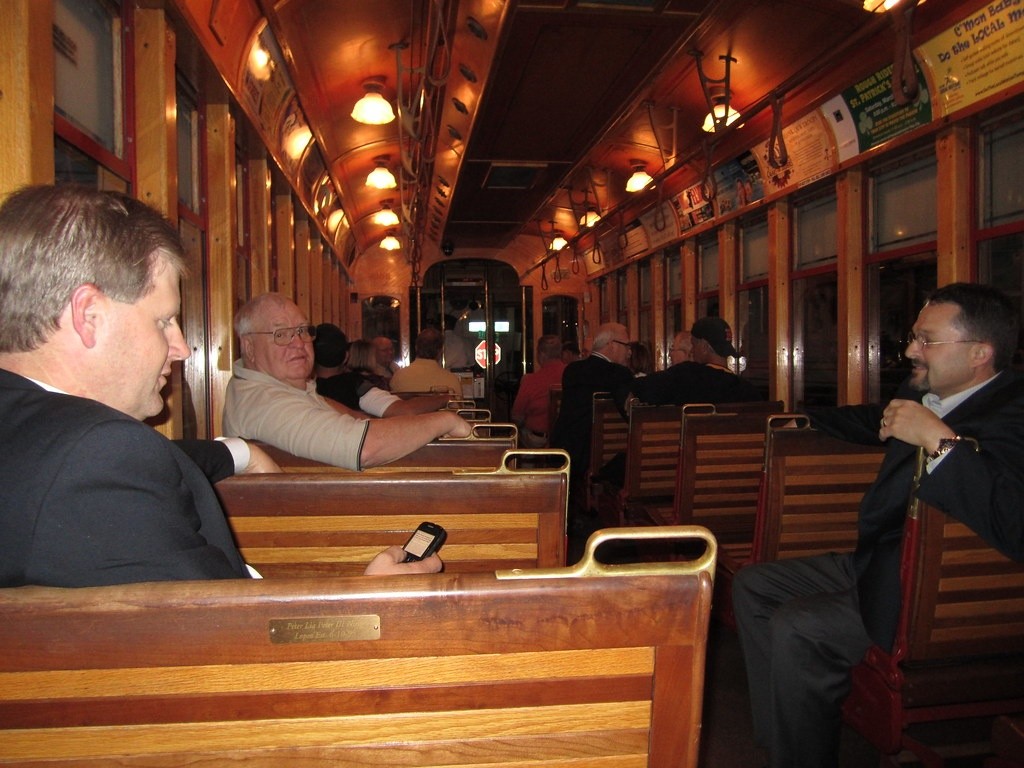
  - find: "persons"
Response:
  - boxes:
[372,336,399,379]
[733,281,1024,768]
[345,339,388,391]
[511,314,762,522]
[309,322,460,420]
[389,328,463,408]
[221,293,479,472]
[0,184,443,590]
[433,312,483,374]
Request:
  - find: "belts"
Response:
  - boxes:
[531,431,548,437]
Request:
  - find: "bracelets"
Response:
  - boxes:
[925,434,962,464]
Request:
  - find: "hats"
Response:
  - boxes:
[690,317,736,357]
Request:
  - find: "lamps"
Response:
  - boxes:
[351,74,402,250]
[547,86,748,250]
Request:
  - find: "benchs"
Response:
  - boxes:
[0,393,1024,768]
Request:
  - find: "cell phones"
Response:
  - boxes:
[398,521,447,564]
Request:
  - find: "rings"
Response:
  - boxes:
[883,419,887,427]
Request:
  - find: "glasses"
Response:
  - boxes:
[244,326,317,347]
[607,339,632,350]
[669,347,682,353]
[906,332,995,361]
